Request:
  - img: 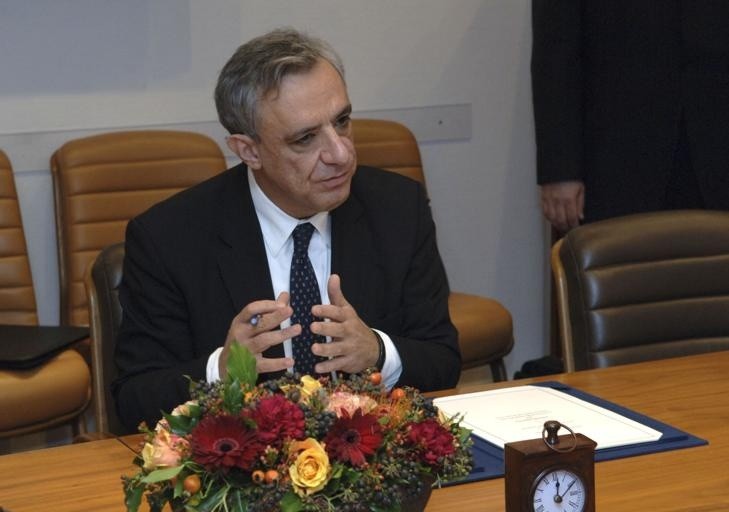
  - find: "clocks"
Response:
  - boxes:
[504,420,598,511]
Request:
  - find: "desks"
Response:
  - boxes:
[0,348,728,509]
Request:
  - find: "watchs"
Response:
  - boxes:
[358,328,386,379]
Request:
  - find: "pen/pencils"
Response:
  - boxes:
[250,313,263,326]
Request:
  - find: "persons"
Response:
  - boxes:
[531,0,727,238]
[112,27,461,431]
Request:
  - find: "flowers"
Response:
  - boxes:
[120,337,477,512]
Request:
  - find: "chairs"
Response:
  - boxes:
[547,210,727,373]
[80,238,145,442]
[50,128,230,443]
[349,118,513,382]
[0,146,94,457]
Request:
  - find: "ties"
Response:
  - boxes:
[289,222,334,380]
